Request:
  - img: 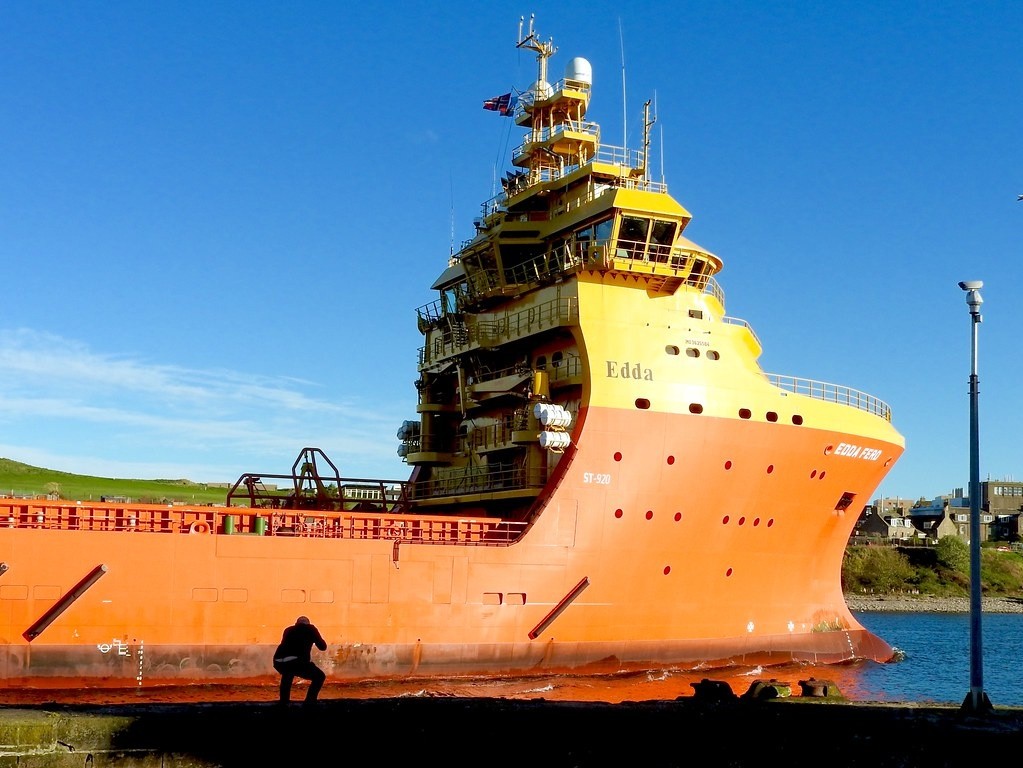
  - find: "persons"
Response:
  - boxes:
[272,616,327,701]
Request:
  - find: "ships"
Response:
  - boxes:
[1,13,904,708]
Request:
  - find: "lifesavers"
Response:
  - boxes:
[388,524,403,539]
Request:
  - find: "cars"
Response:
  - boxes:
[995,546,1011,553]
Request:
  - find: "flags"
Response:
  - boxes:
[483,92,518,118]
